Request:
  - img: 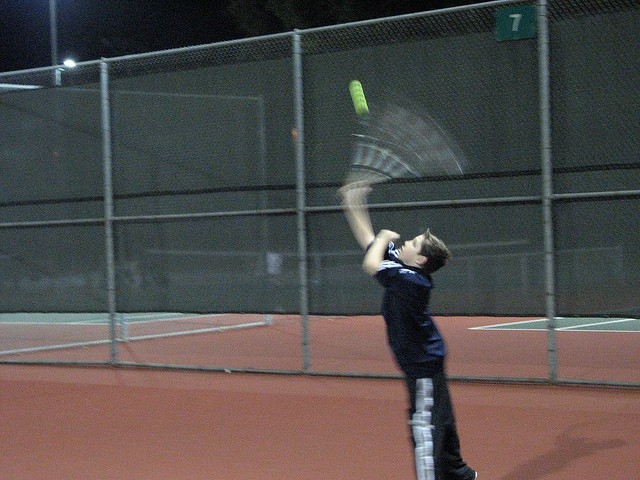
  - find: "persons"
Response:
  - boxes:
[341,179,478,480]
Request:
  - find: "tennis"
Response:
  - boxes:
[348,79,368,114]
